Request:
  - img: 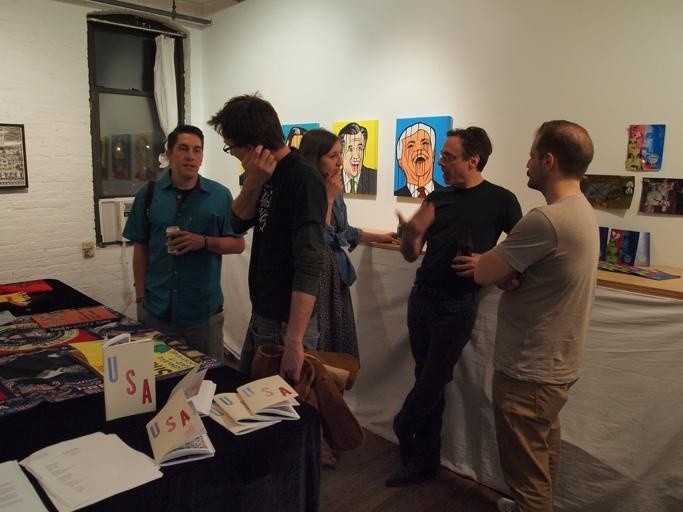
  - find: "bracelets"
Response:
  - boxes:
[201,233,209,250]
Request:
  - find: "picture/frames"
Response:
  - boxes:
[0,123,28,189]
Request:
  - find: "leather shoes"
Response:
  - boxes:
[385,413,438,487]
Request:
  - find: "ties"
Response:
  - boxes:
[349,178,356,192]
[416,186,426,198]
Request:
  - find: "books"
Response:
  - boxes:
[146,361,301,466]
[1,280,216,420]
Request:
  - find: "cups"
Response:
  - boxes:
[165,225,182,255]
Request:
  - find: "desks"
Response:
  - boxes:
[0,279,324,512]
[222,230,683,511]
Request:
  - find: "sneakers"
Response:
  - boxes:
[498,497,517,512]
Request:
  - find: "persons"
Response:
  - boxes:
[283,127,306,150]
[298,127,399,463]
[395,121,446,198]
[116,121,244,364]
[474,120,600,510]
[379,125,525,489]
[335,122,376,195]
[625,126,662,172]
[205,90,328,388]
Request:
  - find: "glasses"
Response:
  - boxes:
[223,144,238,153]
[439,151,474,161]
